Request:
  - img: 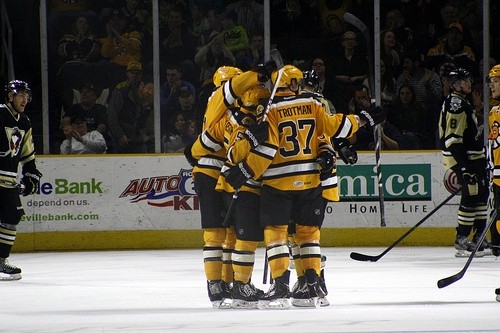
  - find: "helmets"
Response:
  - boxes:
[488,64,500,78]
[271,66,319,87]
[233,84,270,114]
[5,79,32,104]
[212,66,243,90]
[447,67,471,87]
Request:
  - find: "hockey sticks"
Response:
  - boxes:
[349,182,468,263]
[221,48,285,228]
[262,247,274,285]
[437,208,497,289]
[0,179,26,190]
[344,11,387,227]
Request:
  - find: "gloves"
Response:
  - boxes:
[223,160,255,190]
[20,170,43,197]
[243,121,270,150]
[253,60,277,83]
[315,103,386,181]
[184,144,198,166]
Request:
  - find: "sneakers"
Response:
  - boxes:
[207,270,329,310]
[454,235,494,257]
[0,258,21,280]
[495,288,500,302]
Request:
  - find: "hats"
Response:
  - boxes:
[79,80,102,98]
[448,23,463,37]
[399,51,417,63]
[178,83,196,98]
[127,61,142,70]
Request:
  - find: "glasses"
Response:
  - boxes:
[312,63,325,67]
[342,37,354,41]
[16,94,30,99]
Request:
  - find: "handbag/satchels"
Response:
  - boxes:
[211,40,236,67]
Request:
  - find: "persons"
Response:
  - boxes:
[184,63,385,312]
[438,64,500,259]
[284,0,495,150]
[46,0,277,155]
[0,79,42,281]
